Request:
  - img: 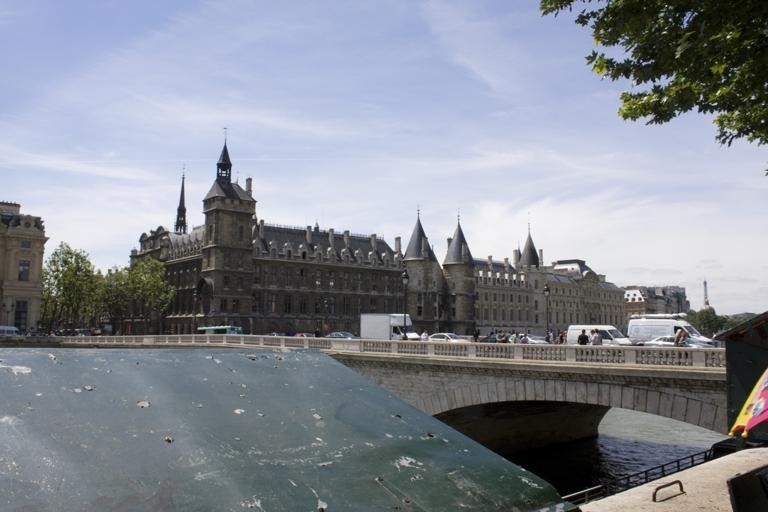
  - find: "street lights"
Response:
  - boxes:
[401,270,409,339]
[543,284,551,341]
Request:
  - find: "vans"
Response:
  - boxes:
[0,326,20,336]
[567,325,631,353]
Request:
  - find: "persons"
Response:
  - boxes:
[472,326,603,349]
[672,329,689,345]
[421,329,429,341]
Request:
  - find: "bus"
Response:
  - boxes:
[197,325,242,335]
[50,329,90,335]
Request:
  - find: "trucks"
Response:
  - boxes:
[359,314,421,341]
[628,318,719,357]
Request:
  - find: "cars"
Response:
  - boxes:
[480,333,549,352]
[644,336,713,355]
[294,334,316,337]
[428,333,471,343]
[326,331,359,339]
